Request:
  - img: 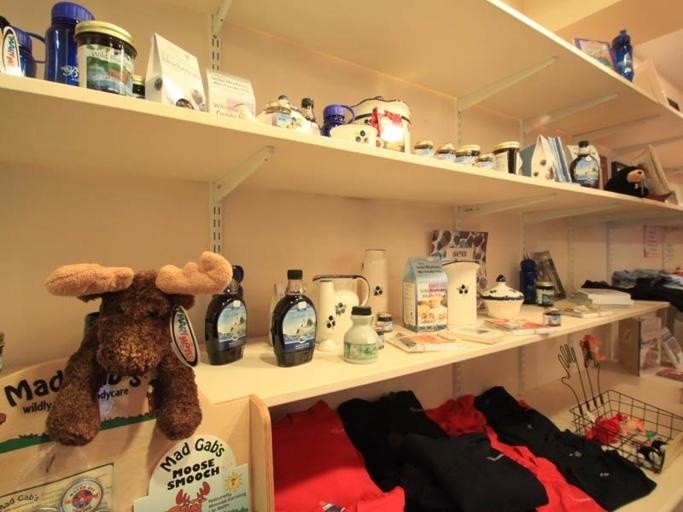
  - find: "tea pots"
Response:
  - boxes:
[311,272,370,351]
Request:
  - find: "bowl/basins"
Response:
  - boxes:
[479,274,526,320]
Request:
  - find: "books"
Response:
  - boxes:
[576,286,634,308]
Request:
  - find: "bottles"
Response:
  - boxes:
[204,266,245,366]
[360,248,387,317]
[270,269,321,366]
[570,141,599,188]
[7,23,50,77]
[42,1,94,87]
[519,260,538,304]
[612,28,632,81]
[321,105,356,137]
[301,98,316,122]
[342,306,378,364]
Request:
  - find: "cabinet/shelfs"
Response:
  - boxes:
[0,1,683,512]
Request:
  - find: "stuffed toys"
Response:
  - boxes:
[40,248,235,448]
[603,165,651,199]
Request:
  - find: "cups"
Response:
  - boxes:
[328,124,377,147]
[440,260,480,323]
